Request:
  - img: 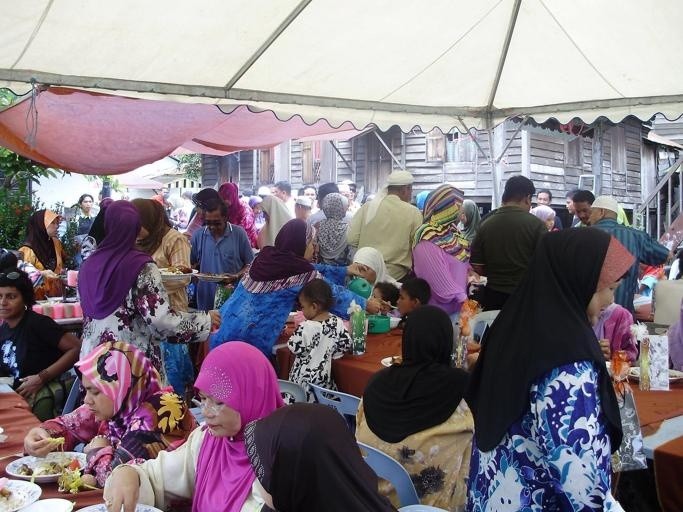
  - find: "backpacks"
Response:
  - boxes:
[23,376,65,421]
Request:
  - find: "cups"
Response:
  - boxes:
[208,309,220,333]
[33,270,85,319]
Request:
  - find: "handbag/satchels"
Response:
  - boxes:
[602,380,650,475]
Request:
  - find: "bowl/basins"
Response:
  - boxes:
[605,361,630,381]
[390,317,401,329]
[286,312,297,322]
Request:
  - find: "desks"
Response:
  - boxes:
[608,375,683,428]
[47,318,85,339]
[623,417,683,512]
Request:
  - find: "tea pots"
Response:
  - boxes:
[347,273,371,299]
[369,314,390,333]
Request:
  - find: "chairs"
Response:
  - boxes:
[276,379,308,405]
[394,505,450,512]
[63,375,82,414]
[356,438,421,503]
[302,380,361,434]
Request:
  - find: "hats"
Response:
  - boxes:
[295,196,313,207]
[590,195,619,214]
[386,169,414,187]
[341,179,357,187]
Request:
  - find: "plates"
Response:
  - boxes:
[381,356,402,367]
[629,366,682,383]
[194,274,228,282]
[0,451,163,512]
[158,267,199,279]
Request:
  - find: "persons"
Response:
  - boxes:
[2,169,683,512]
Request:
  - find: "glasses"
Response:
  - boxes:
[0,271,20,280]
[203,217,227,227]
[190,390,228,417]
